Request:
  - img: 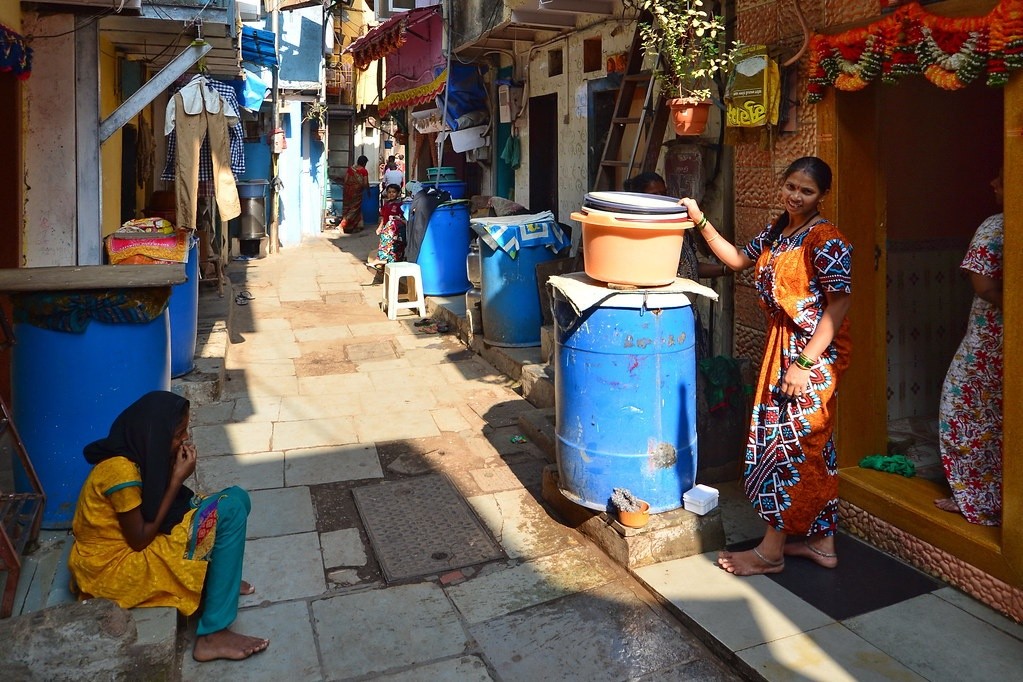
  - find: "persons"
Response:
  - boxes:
[676,157,852,576]
[934,161,1003,527]
[623,172,733,468]
[379,152,405,209]
[390,180,422,225]
[336,155,370,233]
[365,184,402,272]
[68,392,270,661]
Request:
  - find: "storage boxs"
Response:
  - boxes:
[682,483,721,517]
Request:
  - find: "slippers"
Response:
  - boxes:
[437,322,449,333]
[414,319,436,327]
[232,255,257,261]
[235,295,248,305]
[240,291,255,300]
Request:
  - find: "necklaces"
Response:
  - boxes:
[784,211,821,238]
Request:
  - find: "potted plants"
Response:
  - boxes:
[636,0,747,136]
[301,101,329,141]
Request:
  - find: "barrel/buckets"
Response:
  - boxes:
[404,204,469,298]
[553,288,699,517]
[10,238,200,530]
[480,236,554,348]
[232,197,267,241]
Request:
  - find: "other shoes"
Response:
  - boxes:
[419,324,438,334]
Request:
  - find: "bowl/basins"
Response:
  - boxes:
[618,501,651,529]
[571,190,696,286]
[236,182,269,197]
[422,167,467,198]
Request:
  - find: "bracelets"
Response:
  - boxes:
[722,264,727,274]
[706,233,719,242]
[697,211,708,230]
[795,353,814,369]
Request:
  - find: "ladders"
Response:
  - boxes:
[570,5,688,273]
[321,111,353,232]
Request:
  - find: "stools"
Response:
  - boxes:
[381,261,426,320]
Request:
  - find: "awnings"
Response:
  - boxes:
[341,4,442,68]
[242,22,280,126]
